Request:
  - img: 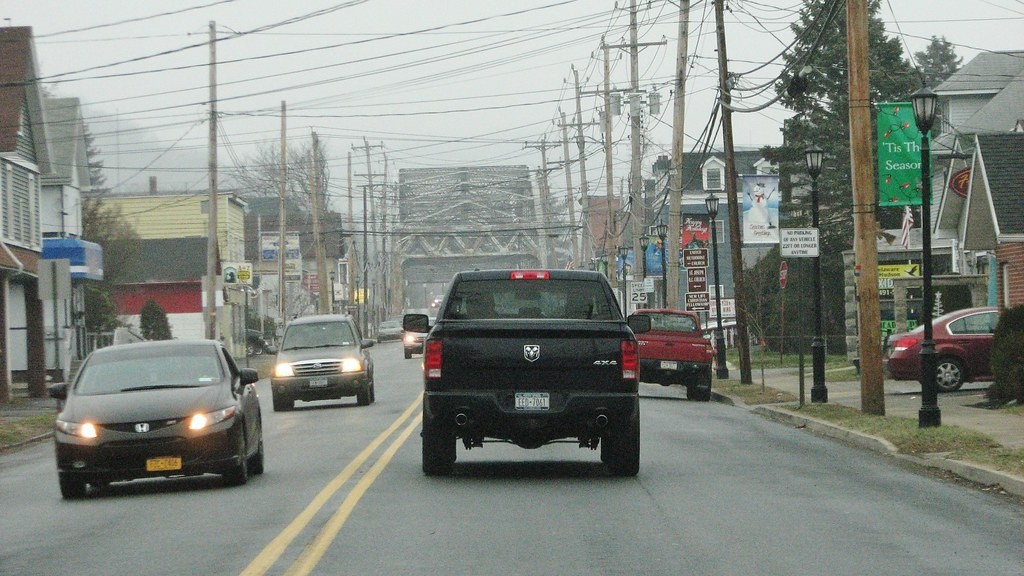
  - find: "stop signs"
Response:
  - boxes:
[778,261,789,287]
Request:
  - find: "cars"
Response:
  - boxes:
[888,305,1000,396]
[402,315,436,358]
[377,320,404,343]
[625,308,714,402]
[44,339,264,500]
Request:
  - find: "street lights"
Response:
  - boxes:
[656,218,668,309]
[618,242,629,322]
[802,144,829,404]
[908,80,942,430]
[637,232,649,307]
[601,253,609,280]
[705,191,730,380]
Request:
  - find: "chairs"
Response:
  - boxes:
[561,292,595,320]
[463,292,500,320]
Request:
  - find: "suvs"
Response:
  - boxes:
[402,268,653,479]
[266,312,377,412]
